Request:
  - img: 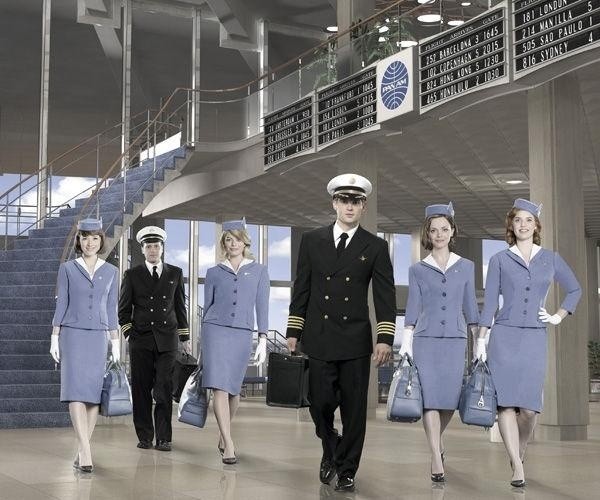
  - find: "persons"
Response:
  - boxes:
[399,203,480,482]
[201,221,270,465]
[286,174,396,492]
[117,225,189,451]
[475,199,582,488]
[50,218,120,472]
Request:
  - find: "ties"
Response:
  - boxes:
[152,265,159,280]
[337,232,350,251]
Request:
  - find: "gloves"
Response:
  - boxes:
[537,306,561,326]
[399,328,415,361]
[252,336,268,367]
[110,337,122,362]
[473,337,490,363]
[49,334,61,364]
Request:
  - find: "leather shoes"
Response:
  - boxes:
[137,439,152,450]
[429,448,447,483]
[72,450,93,473]
[334,476,355,492]
[217,440,236,456]
[509,453,526,488]
[222,450,237,466]
[154,437,173,452]
[319,433,342,485]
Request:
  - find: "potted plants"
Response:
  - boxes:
[588,340,600,393]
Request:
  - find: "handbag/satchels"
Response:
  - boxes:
[458,354,496,429]
[385,352,424,424]
[98,352,134,417]
[264,349,312,408]
[177,362,212,428]
[170,348,198,403]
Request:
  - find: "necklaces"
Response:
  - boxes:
[86,264,95,269]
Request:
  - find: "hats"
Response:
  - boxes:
[76,217,103,232]
[326,172,373,199]
[221,220,247,232]
[512,197,543,218]
[135,225,168,244]
[424,202,456,218]
